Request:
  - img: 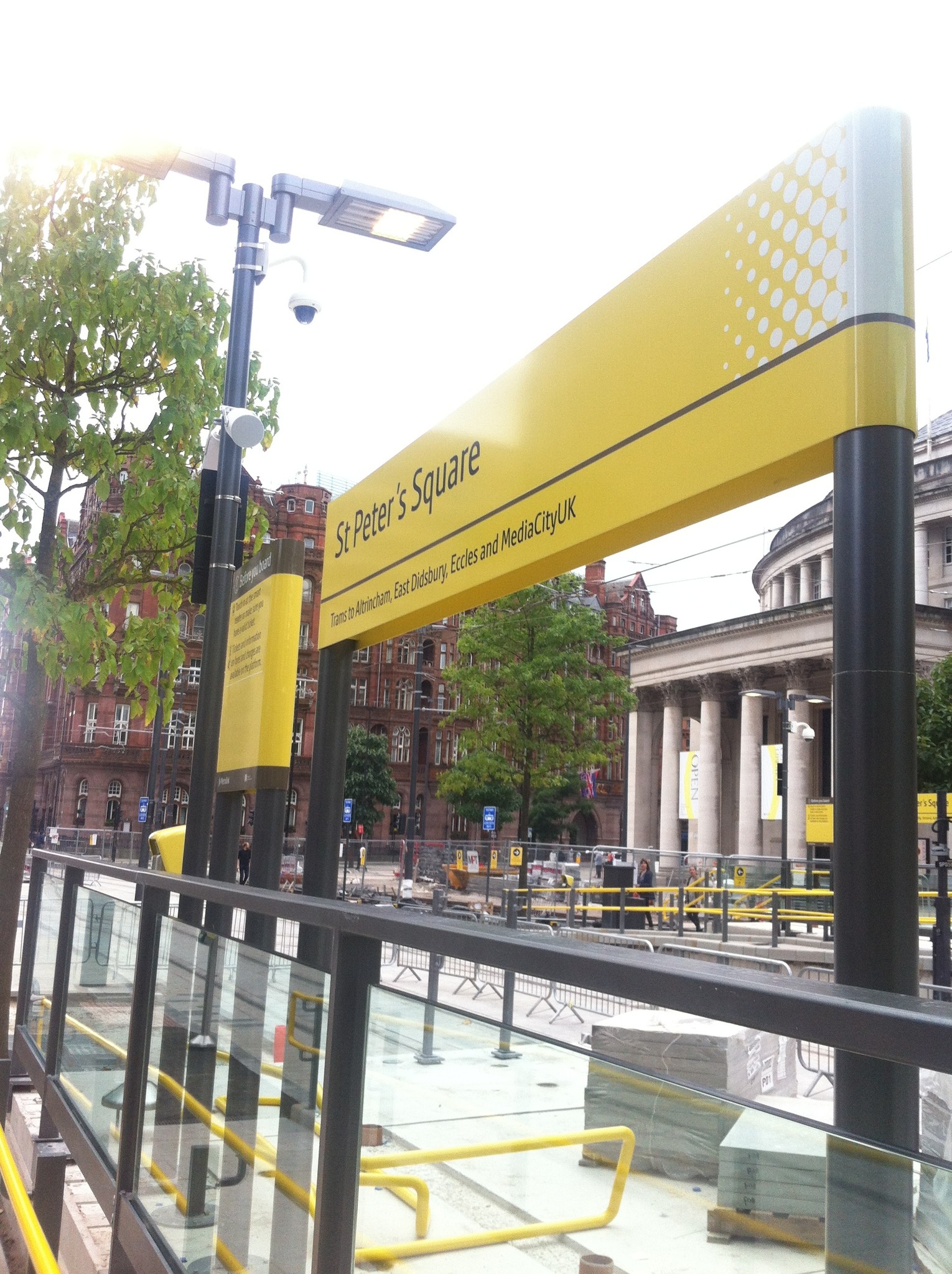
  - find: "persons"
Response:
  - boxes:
[684,867,704,932]
[549,849,565,862]
[238,842,251,885]
[607,851,613,862]
[636,858,655,930]
[36,831,44,847]
[592,852,602,878]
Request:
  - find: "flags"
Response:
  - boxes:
[578,770,598,798]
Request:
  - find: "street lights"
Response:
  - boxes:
[85,129,458,1182]
[740,688,832,935]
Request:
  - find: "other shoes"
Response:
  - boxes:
[645,926,654,930]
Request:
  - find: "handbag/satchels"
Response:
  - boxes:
[39,844,44,848]
[632,892,640,898]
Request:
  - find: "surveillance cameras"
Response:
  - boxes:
[288,292,318,325]
[801,728,815,743]
